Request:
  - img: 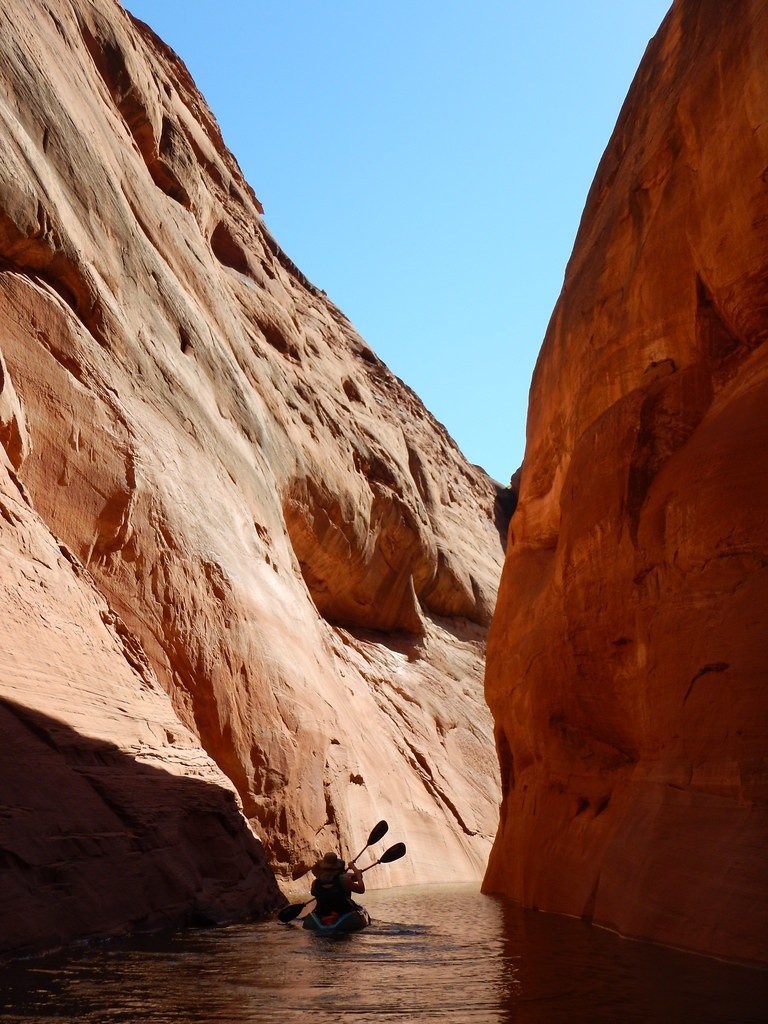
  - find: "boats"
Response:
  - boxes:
[301,908,370,934]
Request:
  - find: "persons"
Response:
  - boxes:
[310,852,365,921]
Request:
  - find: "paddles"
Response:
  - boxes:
[278,819,407,923]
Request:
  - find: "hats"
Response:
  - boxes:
[319,853,344,869]
